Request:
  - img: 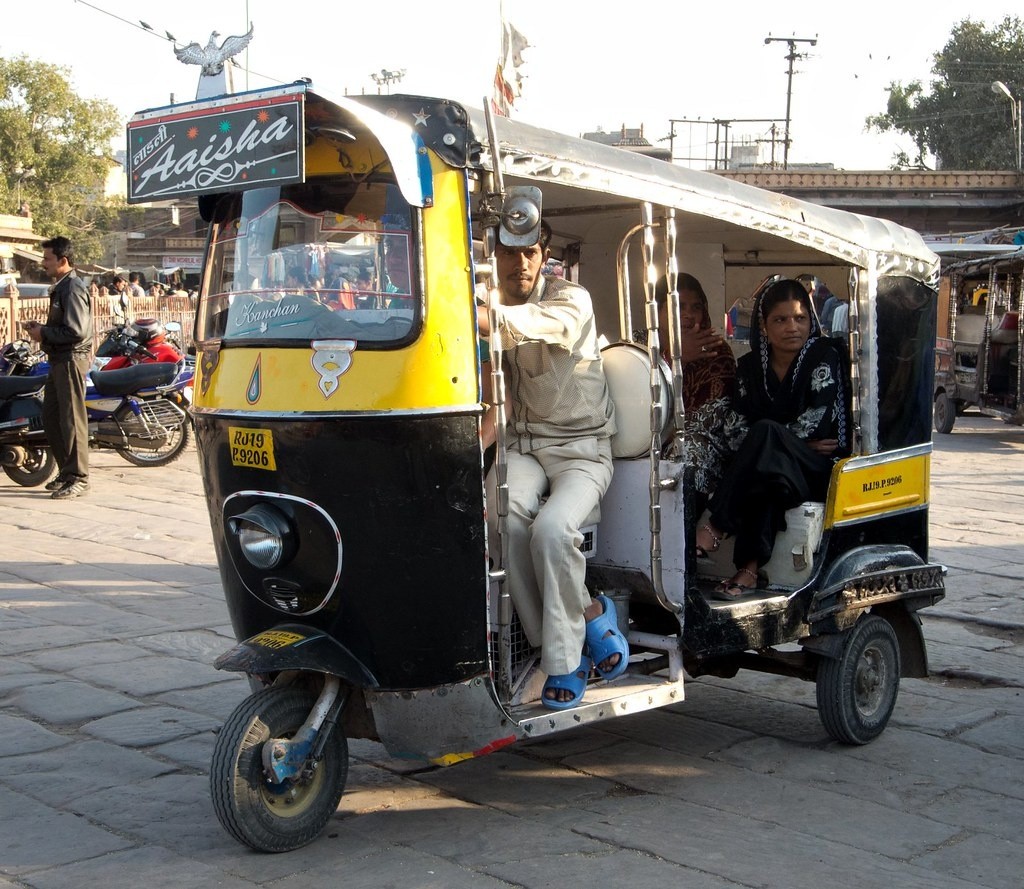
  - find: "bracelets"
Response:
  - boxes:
[662,350,670,366]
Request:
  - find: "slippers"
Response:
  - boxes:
[541,645,592,709]
[585,595,629,680]
[696,545,720,568]
[711,579,757,601]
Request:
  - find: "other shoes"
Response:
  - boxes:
[52,475,89,499]
[45,470,66,490]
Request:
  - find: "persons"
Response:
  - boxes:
[384,220,507,482]
[821,294,851,344]
[87,272,200,296]
[233,268,397,315]
[695,280,854,602]
[622,273,739,593]
[475,218,630,708]
[27,236,93,500]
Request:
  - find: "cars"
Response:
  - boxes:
[0,284,52,299]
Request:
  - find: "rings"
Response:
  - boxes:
[701,346,707,352]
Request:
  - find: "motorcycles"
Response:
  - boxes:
[94,292,196,405]
[0,374,55,487]
[933,249,1024,433]
[126,76,947,854]
[0,339,195,467]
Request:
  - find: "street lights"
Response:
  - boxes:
[764,38,817,170]
[16,169,36,210]
[992,80,1022,170]
